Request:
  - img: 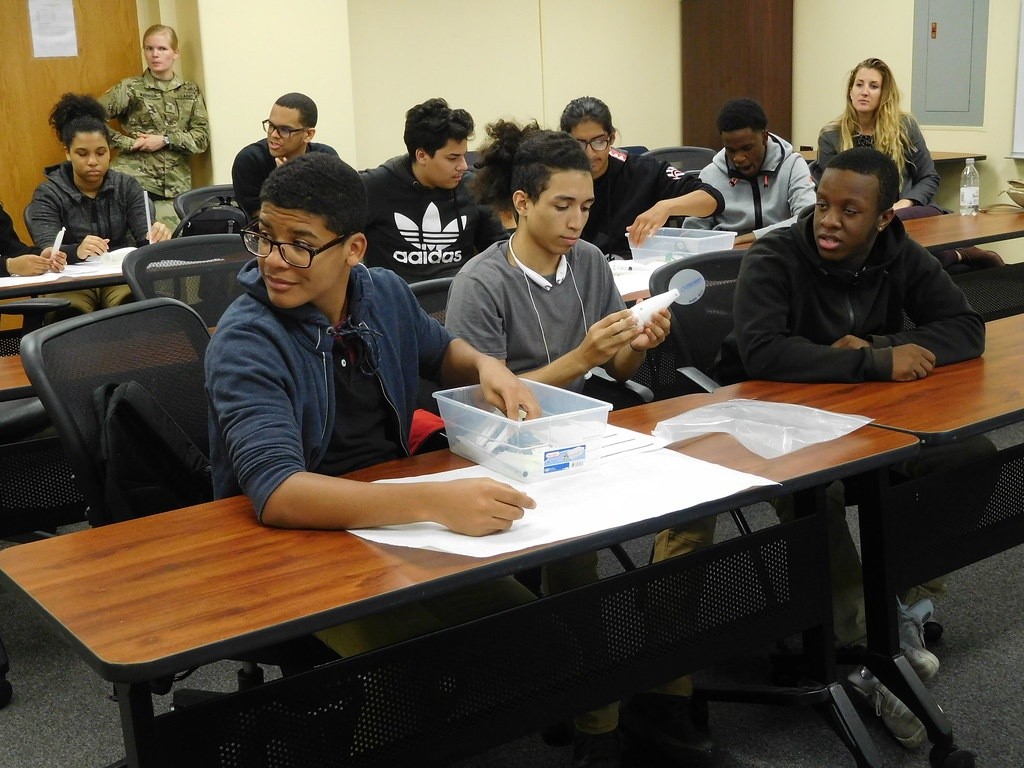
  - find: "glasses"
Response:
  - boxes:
[576,133,611,151]
[262,119,310,138]
[240,218,362,268]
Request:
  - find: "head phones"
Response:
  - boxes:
[509,231,568,291]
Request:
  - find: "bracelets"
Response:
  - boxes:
[7,258,12,275]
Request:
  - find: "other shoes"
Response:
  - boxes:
[618,694,717,767]
[572,727,618,768]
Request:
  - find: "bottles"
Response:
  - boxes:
[960,157,979,217]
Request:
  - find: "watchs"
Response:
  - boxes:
[163,135,170,148]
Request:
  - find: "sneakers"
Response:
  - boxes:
[895,597,940,684]
[846,664,943,749]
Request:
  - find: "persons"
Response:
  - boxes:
[30,91,171,326]
[358,98,511,313]
[204,153,538,755]
[560,97,726,262]
[232,93,339,225]
[720,149,986,748]
[441,118,715,768]
[98,24,209,297]
[0,204,67,277]
[683,99,817,245]
[816,59,953,221]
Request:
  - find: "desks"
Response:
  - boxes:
[1,211,1024,768]
[798,150,988,166]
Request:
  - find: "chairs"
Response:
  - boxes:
[0,145,758,717]
